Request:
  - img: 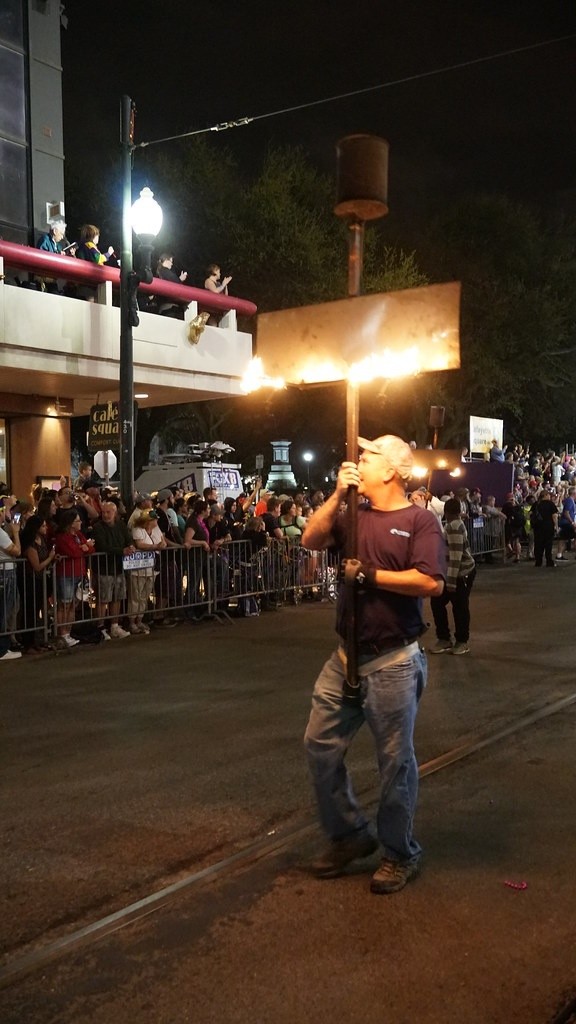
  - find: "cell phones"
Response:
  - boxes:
[52,545,56,551]
[12,513,22,524]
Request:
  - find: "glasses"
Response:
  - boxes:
[74,520,82,523]
[62,491,74,495]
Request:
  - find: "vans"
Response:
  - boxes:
[135,441,246,508]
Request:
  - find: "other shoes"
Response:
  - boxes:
[545,564,558,568]
[528,557,535,561]
[0,615,186,659]
[485,559,495,564]
[555,556,569,561]
[513,559,520,563]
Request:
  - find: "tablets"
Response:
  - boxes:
[63,243,78,255]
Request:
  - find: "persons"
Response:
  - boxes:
[77,224,114,302]
[0,481,576,660]
[204,264,232,327]
[301,434,448,892]
[409,441,416,449]
[429,498,477,654]
[74,463,91,488]
[461,447,468,462]
[491,439,576,488]
[36,220,76,294]
[156,253,188,318]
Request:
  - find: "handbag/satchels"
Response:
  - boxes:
[154,553,160,571]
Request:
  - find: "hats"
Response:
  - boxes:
[260,489,275,497]
[279,494,290,501]
[357,435,413,481]
[211,505,226,515]
[158,489,172,502]
[140,508,161,521]
[137,492,151,503]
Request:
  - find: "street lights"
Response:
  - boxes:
[114,93,167,547]
[302,450,314,500]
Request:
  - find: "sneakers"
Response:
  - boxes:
[429,640,453,653]
[453,641,470,654]
[307,834,378,875]
[370,849,418,892]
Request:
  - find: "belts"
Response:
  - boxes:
[346,638,414,654]
